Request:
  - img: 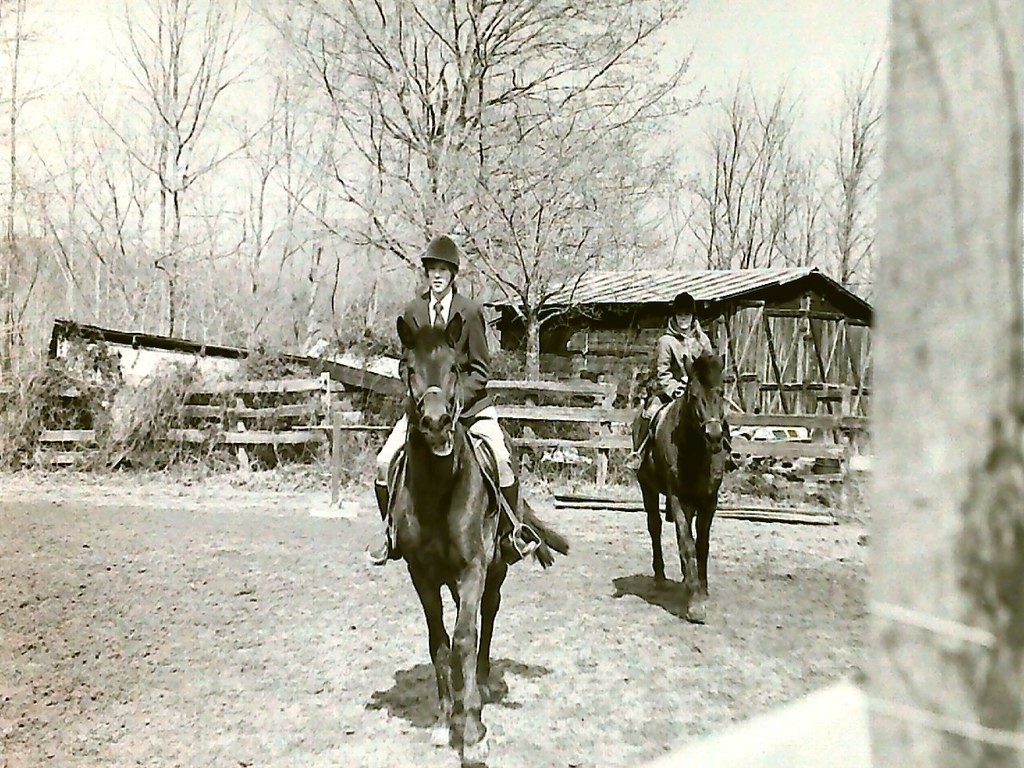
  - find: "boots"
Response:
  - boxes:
[629,413,650,468]
[374,480,402,561]
[500,476,537,564]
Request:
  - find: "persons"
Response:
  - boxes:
[625,292,742,470]
[375,237,538,559]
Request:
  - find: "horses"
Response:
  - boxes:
[629,349,727,625]
[392,311,508,767]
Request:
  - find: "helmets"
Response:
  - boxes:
[673,292,696,315]
[420,235,460,273]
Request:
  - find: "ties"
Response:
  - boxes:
[434,303,445,329]
